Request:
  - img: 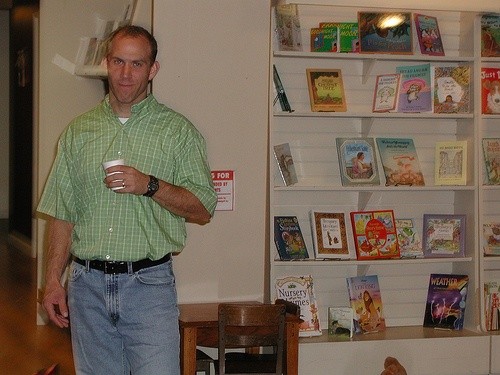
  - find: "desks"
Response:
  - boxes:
[180,300,304,375]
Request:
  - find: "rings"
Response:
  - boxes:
[122,181,124,187]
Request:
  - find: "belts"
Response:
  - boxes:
[68,250,172,275]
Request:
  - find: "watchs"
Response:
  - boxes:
[143,175,159,197]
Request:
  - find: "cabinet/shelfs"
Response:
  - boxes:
[264,0,500,375]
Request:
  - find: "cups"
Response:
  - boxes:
[102,159,127,191]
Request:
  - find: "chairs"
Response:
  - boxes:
[214,303,286,375]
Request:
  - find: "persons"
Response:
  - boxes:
[37,25,217,375]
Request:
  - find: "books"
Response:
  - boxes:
[272,4,500,339]
